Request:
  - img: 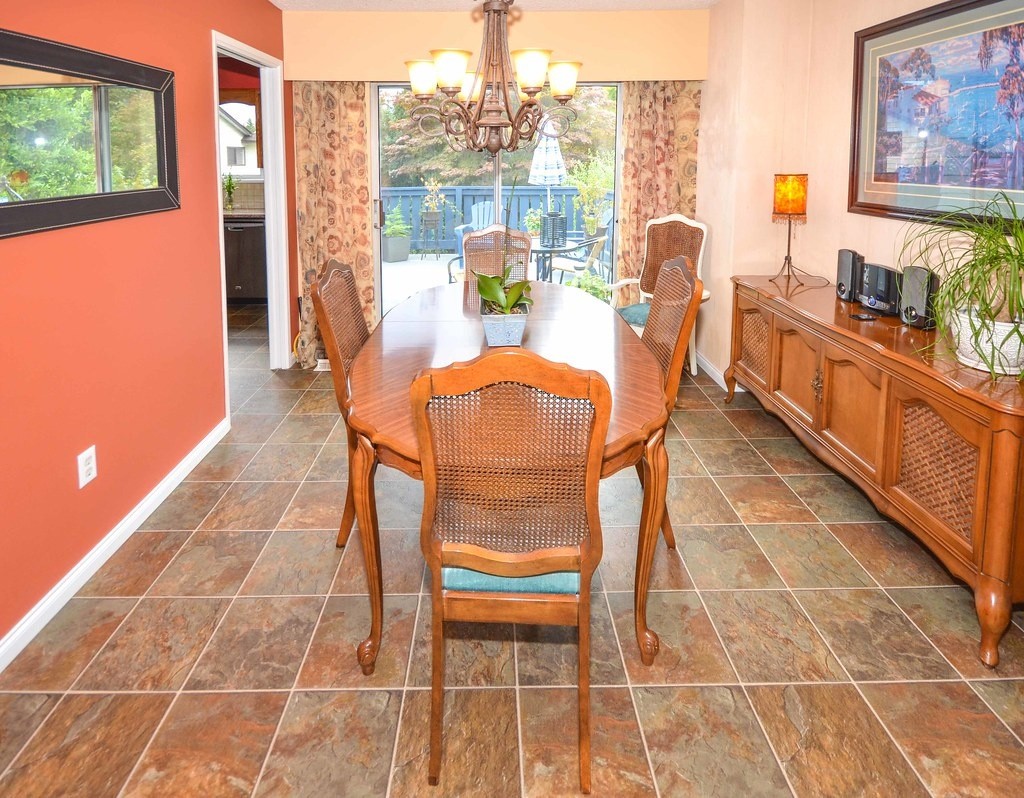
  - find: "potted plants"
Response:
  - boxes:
[382,195,413,262]
[896,188,1024,385]
[469,169,534,347]
[567,156,614,252]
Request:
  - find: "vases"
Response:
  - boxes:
[422,212,440,221]
[224,194,234,211]
[527,230,539,236]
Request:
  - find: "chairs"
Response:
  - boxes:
[410,346,611,795]
[604,212,712,376]
[455,201,609,288]
[310,259,369,548]
[633,255,703,548]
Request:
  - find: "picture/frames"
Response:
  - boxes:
[845,0,1024,235]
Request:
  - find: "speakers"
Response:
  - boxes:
[836,249,864,301]
[900,265,940,329]
[857,261,903,316]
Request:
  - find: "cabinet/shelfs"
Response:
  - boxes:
[724,274,1024,666]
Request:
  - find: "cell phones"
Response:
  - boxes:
[849,314,876,320]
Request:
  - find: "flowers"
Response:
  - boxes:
[523,207,542,230]
[220,170,239,196]
[419,174,448,211]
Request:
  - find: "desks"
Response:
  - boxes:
[343,278,670,675]
[510,237,578,283]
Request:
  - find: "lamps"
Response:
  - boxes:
[769,174,811,287]
[404,0,583,158]
